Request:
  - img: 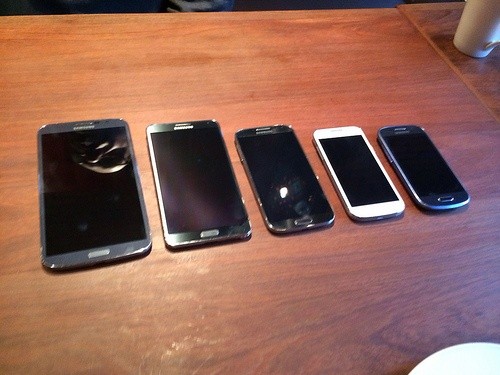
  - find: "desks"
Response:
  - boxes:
[0,7,500,375]
[398,2,500,125]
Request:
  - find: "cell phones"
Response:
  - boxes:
[311,126,405,222]
[147,120,252,249]
[36,118,151,272]
[377,124,471,212]
[233,125,336,235]
